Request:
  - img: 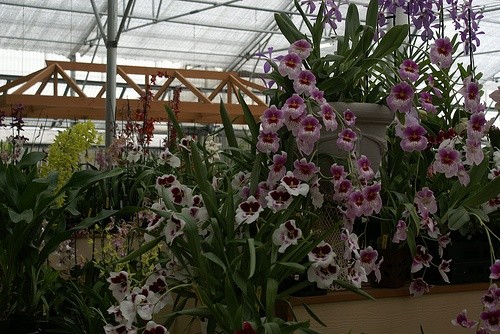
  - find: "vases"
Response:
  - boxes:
[287,256,327,297]
[371,249,408,289]
[292,101,395,182]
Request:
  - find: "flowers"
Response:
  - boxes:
[0,0,500,334]
